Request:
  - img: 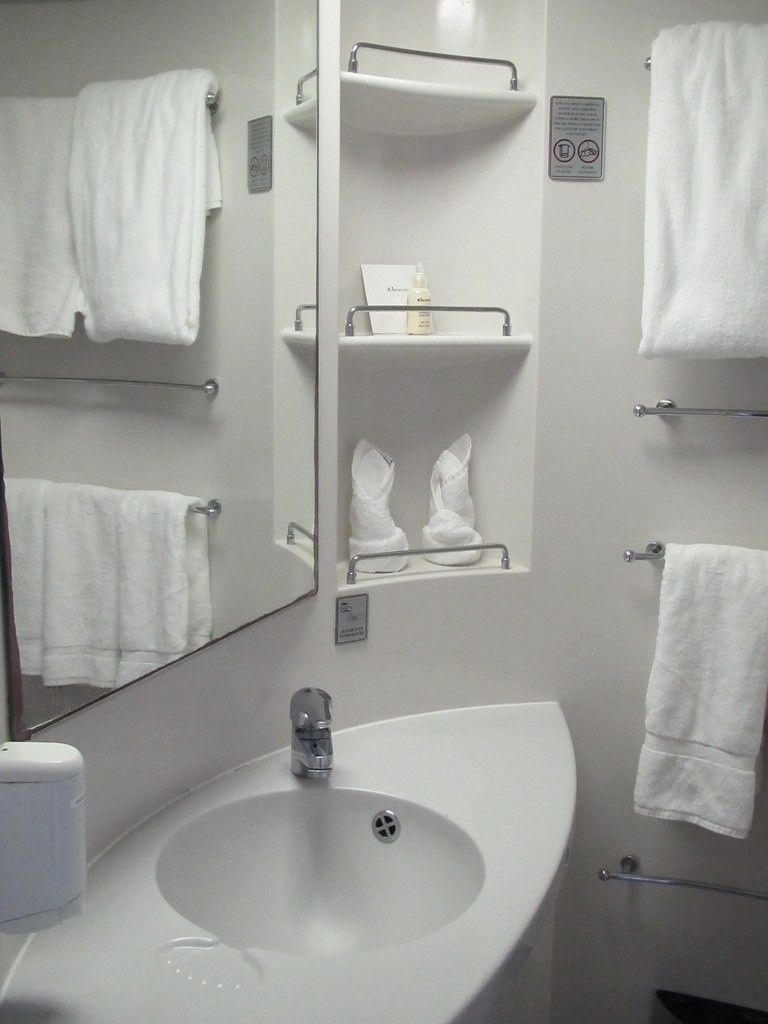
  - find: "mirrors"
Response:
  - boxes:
[0,0,321,743]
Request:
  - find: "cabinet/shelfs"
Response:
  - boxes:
[334,0,547,578]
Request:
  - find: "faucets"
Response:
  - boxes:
[288,685,338,780]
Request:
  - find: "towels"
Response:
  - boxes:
[639,18,768,366]
[81,68,230,347]
[0,92,86,350]
[421,431,484,566]
[630,540,768,842]
[347,433,411,575]
[0,471,218,693]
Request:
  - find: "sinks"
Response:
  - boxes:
[150,774,494,961]
